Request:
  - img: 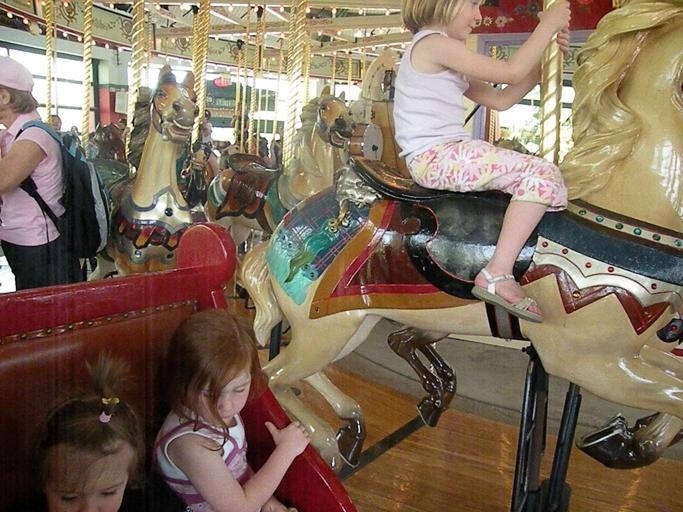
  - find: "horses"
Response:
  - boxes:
[85,64,199,276]
[207,85,357,247]
[241,4,683,477]
[184,140,212,204]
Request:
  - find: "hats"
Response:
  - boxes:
[0,55,34,94]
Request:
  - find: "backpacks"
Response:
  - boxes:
[12,121,110,257]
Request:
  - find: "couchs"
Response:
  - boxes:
[0,222,358,512]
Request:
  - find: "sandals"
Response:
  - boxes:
[471,267,543,324]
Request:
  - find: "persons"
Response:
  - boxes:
[150,308,311,512]
[0,56,73,291]
[50,115,61,131]
[28,347,146,512]
[393,0,572,323]
[181,110,219,182]
